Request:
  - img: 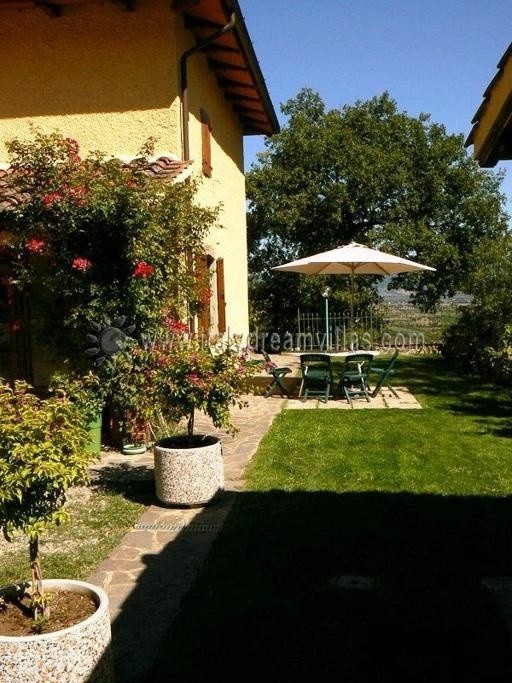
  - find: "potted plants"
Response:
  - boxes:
[0,328,277,682]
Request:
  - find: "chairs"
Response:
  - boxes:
[261,349,400,404]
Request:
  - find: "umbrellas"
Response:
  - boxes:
[270,239,437,350]
